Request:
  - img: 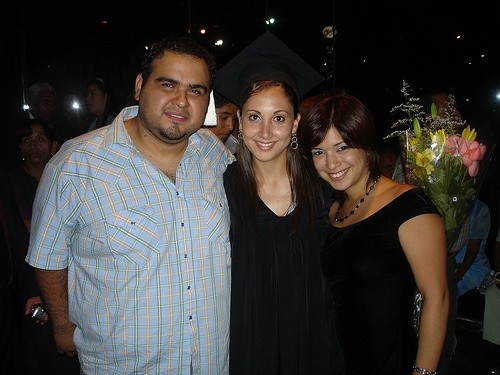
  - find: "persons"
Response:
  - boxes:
[454,198,490,315]
[213,31,344,375]
[25,80,56,123]
[298,92,449,375]
[25,37,231,375]
[206,88,240,143]
[85,74,115,132]
[14,110,58,375]
[391,94,461,184]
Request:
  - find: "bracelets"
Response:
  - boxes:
[413,364,436,375]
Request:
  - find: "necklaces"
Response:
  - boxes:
[334,174,379,222]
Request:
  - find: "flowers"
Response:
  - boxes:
[382,76,486,244]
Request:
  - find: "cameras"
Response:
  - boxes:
[29,304,46,320]
